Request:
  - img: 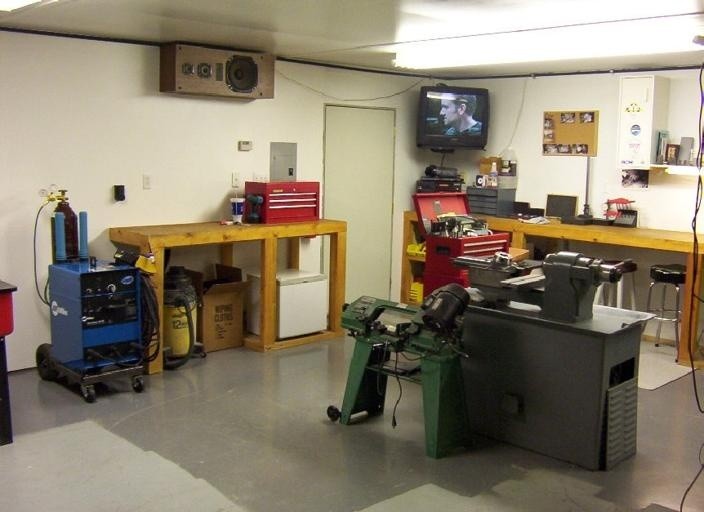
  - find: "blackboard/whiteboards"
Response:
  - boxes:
[544,198,580,219]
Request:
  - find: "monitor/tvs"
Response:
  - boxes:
[417,85,489,150]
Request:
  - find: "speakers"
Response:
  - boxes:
[160,43,274,99]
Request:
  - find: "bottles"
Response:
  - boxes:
[490,162,498,177]
[49,200,80,262]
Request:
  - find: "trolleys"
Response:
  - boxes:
[35,344,146,402]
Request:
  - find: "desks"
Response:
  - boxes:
[106,218,348,376]
[513,209,704,370]
[1,278,18,447]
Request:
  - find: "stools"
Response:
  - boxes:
[641,262,687,362]
[592,259,638,311]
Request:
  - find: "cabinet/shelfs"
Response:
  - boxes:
[397,208,529,311]
[614,74,671,173]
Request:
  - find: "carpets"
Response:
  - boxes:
[634,336,701,393]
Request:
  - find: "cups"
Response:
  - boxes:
[665,144,680,164]
[510,162,516,175]
[229,197,245,224]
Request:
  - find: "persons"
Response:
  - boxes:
[439,94,481,135]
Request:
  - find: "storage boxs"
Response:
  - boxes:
[244,268,330,341]
[185,263,254,354]
